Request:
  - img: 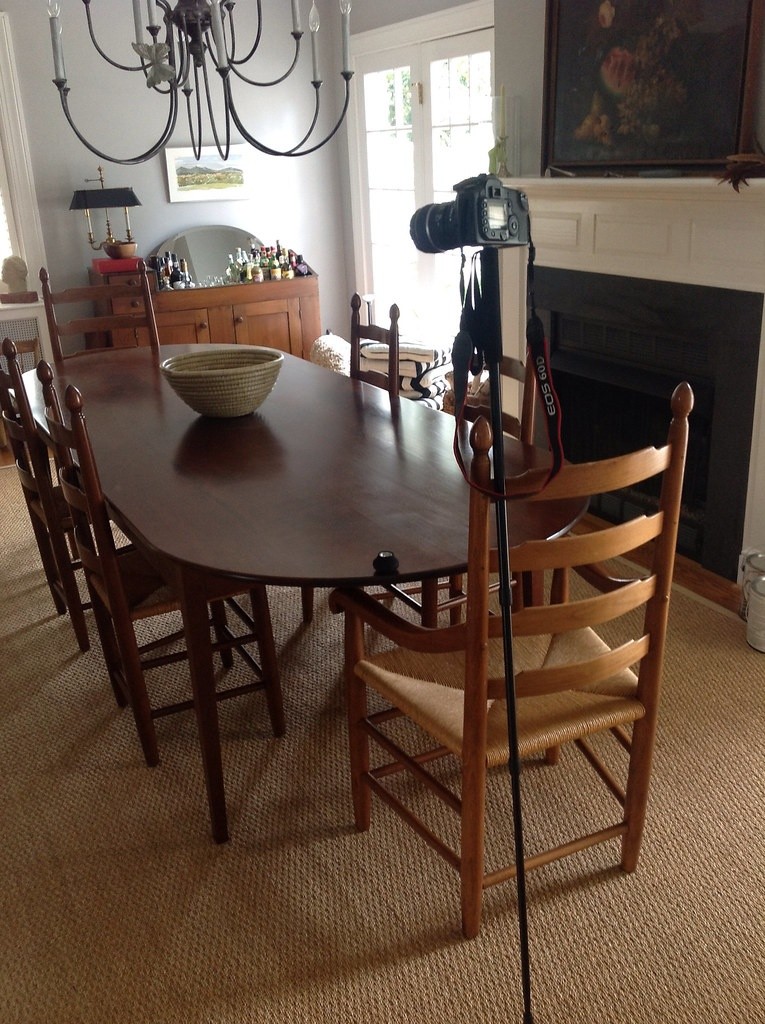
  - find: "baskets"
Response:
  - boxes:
[159,348,284,417]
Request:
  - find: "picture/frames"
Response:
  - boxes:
[540,0,765,179]
[164,144,251,203]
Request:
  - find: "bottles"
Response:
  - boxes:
[226,240,307,284]
[150,252,188,290]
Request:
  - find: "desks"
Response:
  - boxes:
[7,344,590,843]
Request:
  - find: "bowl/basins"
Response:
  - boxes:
[159,349,284,417]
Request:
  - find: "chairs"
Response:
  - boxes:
[329,382,696,939]
[383,330,548,630]
[349,293,401,394]
[0,337,112,653]
[39,259,161,361]
[36,357,286,765]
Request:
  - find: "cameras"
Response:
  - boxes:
[410,174,528,254]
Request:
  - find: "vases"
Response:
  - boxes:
[103,242,138,259]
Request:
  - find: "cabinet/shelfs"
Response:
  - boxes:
[86,249,321,362]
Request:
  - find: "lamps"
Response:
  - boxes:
[47,0,356,165]
[69,165,142,251]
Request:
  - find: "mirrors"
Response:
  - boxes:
[157,225,264,287]
